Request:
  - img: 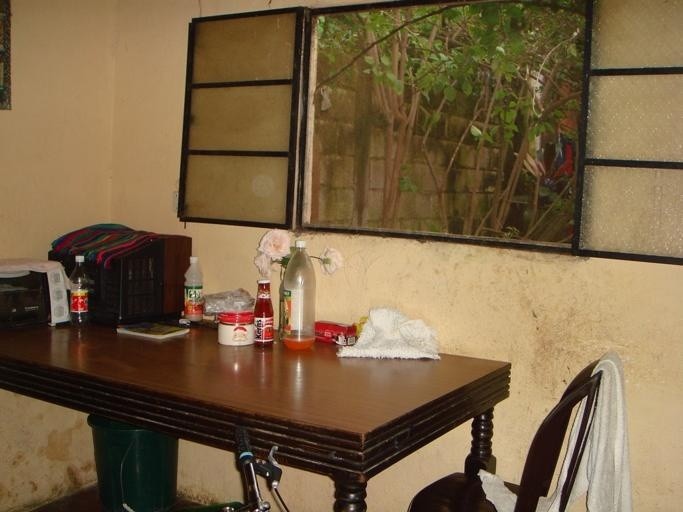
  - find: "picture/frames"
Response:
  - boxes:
[0,0,12,111]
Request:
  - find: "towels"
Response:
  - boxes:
[335,306,443,362]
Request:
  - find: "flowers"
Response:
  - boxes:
[253,227,341,337]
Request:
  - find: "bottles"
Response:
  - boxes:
[283,241,317,350]
[68,256,88,339]
[183,255,204,321]
[254,279,274,347]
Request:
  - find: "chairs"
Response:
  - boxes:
[405,359,603,512]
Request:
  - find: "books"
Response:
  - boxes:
[116,322,190,340]
